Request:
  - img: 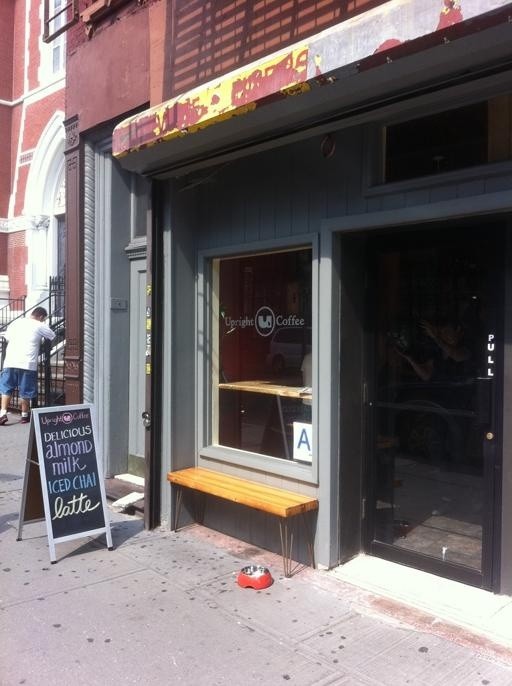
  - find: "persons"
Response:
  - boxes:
[246,307,485,413]
[393,314,476,516]
[1,306,58,424]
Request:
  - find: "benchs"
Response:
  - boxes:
[168,465,321,575]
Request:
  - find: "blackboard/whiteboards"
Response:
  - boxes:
[15,404,110,543]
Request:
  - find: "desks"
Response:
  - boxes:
[219,378,314,457]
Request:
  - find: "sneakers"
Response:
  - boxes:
[0,414,8,425]
[21,416,30,423]
